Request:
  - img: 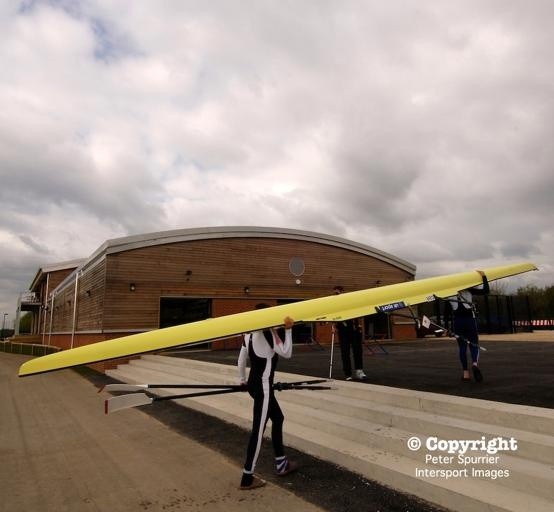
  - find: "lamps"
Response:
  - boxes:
[245,286,249,293]
[130,282,135,291]
[86,290,90,296]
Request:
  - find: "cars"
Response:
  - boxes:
[419,313,453,338]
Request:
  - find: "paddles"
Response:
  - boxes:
[105,385,331,415]
[98,379,328,393]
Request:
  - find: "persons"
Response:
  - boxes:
[447,268,490,383]
[238,304,299,489]
[330,285,367,380]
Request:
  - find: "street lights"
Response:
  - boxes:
[1,313,7,340]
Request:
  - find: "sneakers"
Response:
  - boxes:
[355,369,367,380]
[345,376,352,381]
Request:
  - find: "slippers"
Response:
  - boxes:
[471,365,484,385]
[276,458,300,477]
[239,473,267,490]
